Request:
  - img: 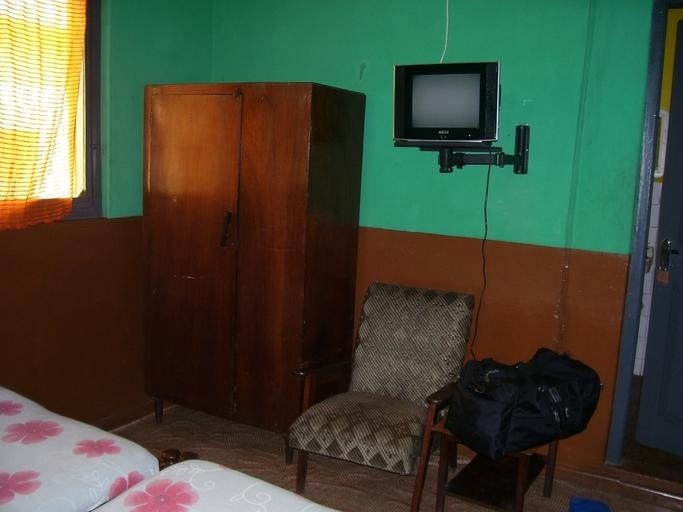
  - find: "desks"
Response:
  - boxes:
[431,414,557,511]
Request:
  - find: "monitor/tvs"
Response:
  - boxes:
[391,60,501,148]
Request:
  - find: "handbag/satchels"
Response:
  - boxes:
[443,344,601,461]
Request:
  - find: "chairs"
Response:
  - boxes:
[285,279,475,512]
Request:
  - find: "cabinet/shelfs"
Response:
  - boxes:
[140,81,366,468]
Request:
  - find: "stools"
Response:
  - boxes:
[1,385,159,512]
[84,458,349,512]
